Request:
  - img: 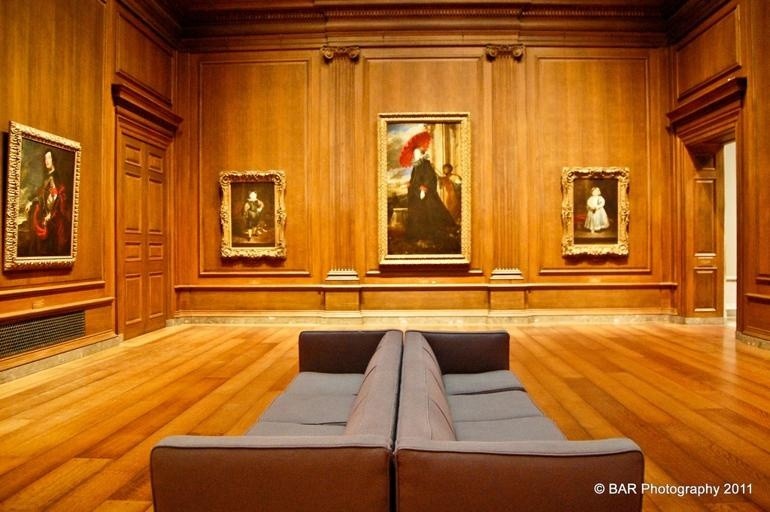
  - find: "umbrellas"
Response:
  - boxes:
[399,131,445,178]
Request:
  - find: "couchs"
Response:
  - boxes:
[147,324,407,512]
[395,328,651,510]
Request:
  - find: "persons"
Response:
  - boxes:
[240,187,267,241]
[404,145,458,249]
[22,148,72,256]
[583,185,610,235]
[437,162,462,224]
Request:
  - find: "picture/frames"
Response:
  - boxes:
[218,165,291,261]
[4,118,82,273]
[559,163,632,263]
[374,110,475,269]
